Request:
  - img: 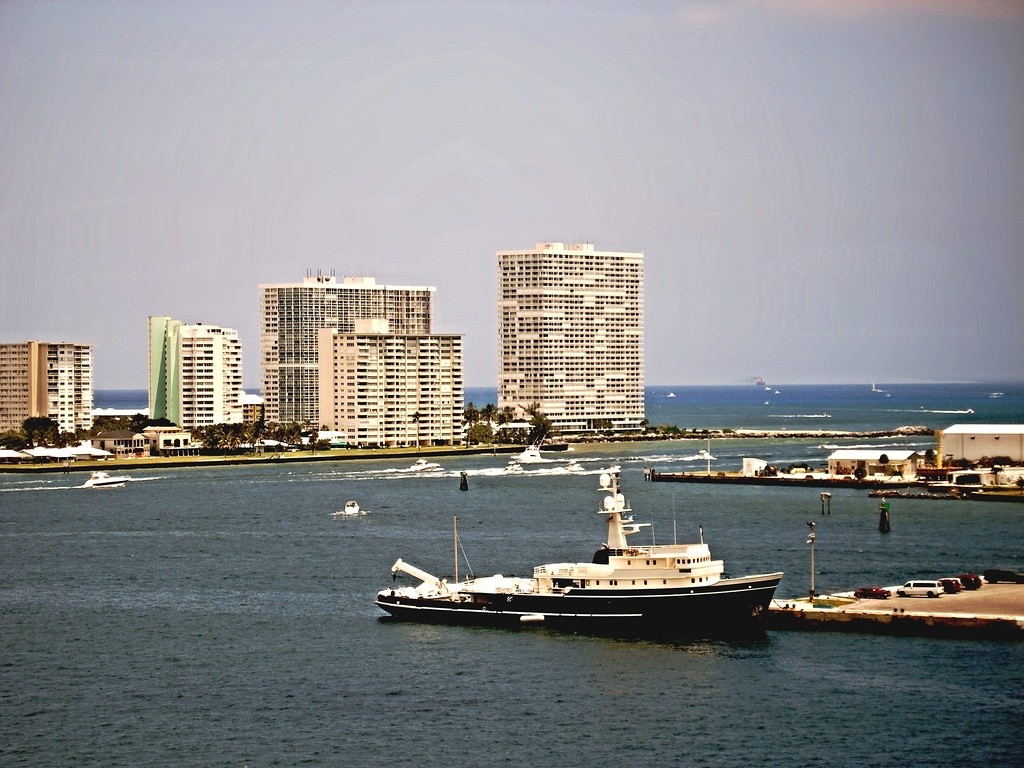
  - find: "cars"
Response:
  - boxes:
[938,573,982,594]
[854,587,892,599]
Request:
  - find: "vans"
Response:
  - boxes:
[897,580,944,598]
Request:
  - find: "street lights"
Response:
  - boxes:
[806,522,816,601]
[906,428,909,451]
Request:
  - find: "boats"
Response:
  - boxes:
[565,461,586,471]
[503,464,523,471]
[410,459,444,472]
[375,467,784,632]
[84,470,131,486]
[511,435,548,460]
[756,376,765,385]
[667,392,676,398]
[699,449,718,460]
[765,387,771,391]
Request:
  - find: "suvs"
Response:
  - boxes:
[983,569,1024,584]
